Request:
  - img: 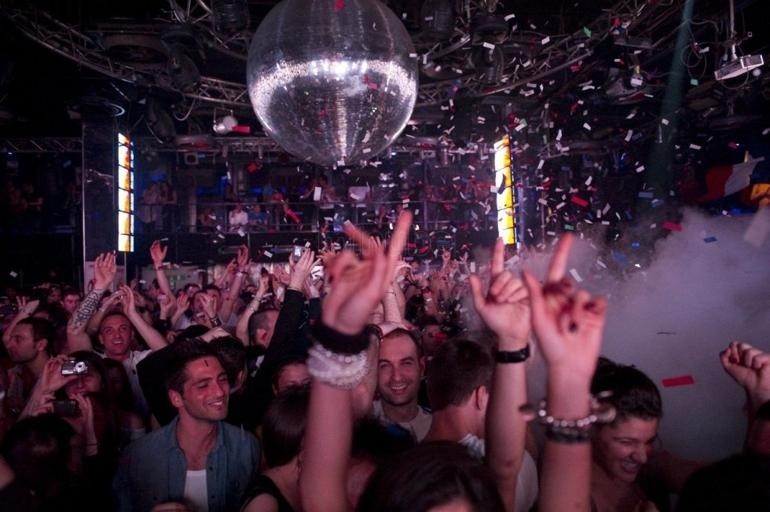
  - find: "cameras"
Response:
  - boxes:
[61,359,89,376]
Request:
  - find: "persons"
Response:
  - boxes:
[130,160,494,260]
[0,207,768,512]
